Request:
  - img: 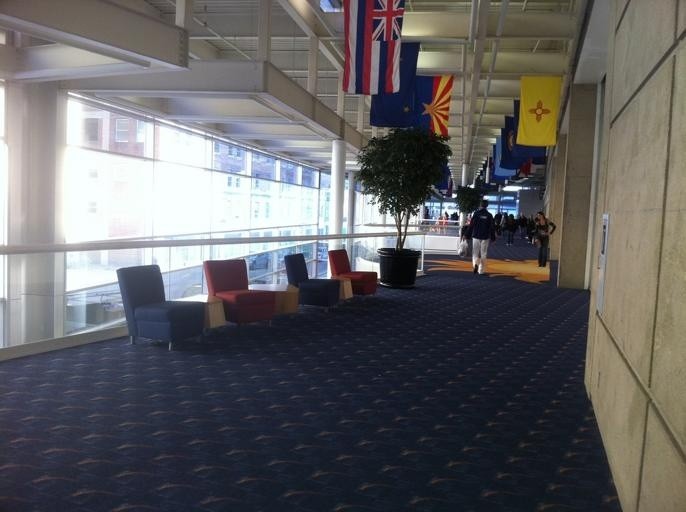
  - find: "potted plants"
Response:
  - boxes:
[354,125,451,291]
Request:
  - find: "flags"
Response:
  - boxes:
[470,99,548,192]
[341,1,405,94]
[438,164,454,200]
[407,73,455,141]
[517,75,564,147]
[369,40,420,130]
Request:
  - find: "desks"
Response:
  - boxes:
[319,276,353,303]
[247,283,301,321]
[172,293,226,332]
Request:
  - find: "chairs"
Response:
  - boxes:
[283,253,340,313]
[116,264,207,349]
[327,248,379,299]
[202,258,275,332]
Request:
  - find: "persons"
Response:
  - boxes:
[424,208,459,235]
[532,211,557,267]
[494,210,535,244]
[462,199,497,276]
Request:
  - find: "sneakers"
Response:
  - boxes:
[479,271,485,275]
[538,264,546,267]
[473,264,478,274]
